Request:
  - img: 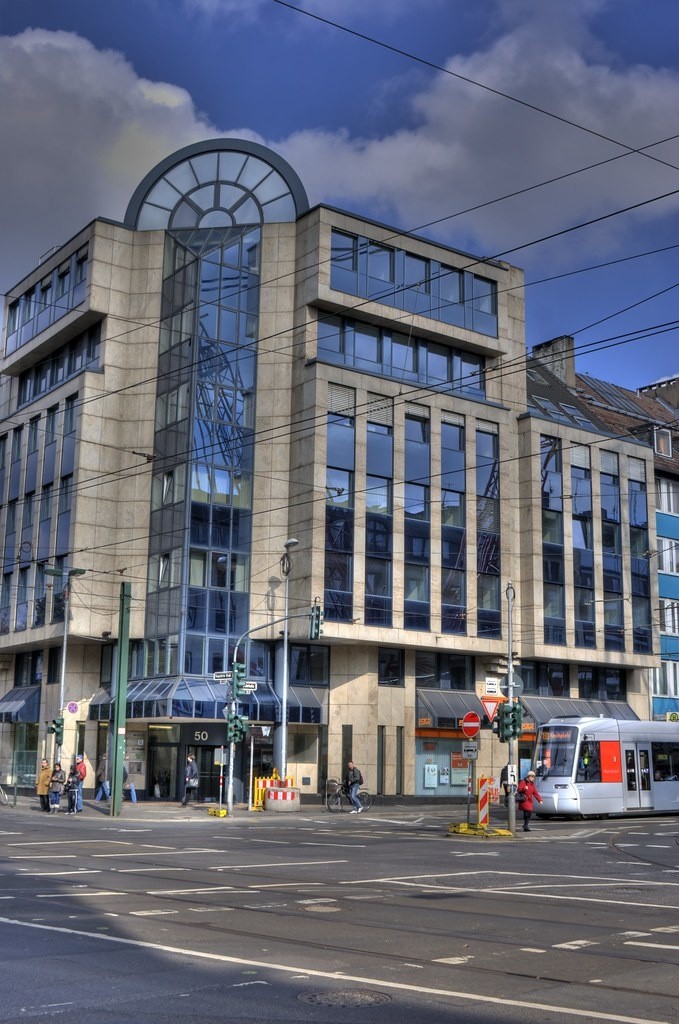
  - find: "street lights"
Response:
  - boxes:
[44,567,86,762]
[282,538,299,779]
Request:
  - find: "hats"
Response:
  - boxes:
[77,754,83,759]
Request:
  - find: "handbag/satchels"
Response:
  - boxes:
[515,792,526,803]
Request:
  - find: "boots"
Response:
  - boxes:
[48,804,59,814]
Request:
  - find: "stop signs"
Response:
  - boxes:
[462,712,481,737]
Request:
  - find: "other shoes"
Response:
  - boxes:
[349,807,363,813]
[65,811,76,814]
[523,825,531,832]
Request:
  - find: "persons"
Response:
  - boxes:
[35,759,52,812]
[95,753,111,803]
[75,755,85,812]
[179,753,200,807]
[64,765,80,816]
[517,771,542,832]
[536,760,547,776]
[347,761,363,813]
[48,762,64,813]
[122,764,127,802]
[500,763,509,807]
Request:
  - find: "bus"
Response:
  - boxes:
[531,714,679,818]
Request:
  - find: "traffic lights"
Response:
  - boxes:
[307,606,324,640]
[498,703,516,744]
[228,714,248,743]
[52,718,63,744]
[492,716,500,734]
[233,664,246,699]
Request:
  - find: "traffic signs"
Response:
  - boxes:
[508,765,517,786]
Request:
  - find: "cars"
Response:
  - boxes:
[17,769,35,785]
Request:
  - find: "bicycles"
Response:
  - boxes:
[0,772,9,806]
[327,783,372,813]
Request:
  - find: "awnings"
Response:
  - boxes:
[517,697,641,734]
[413,689,490,730]
[0,686,40,724]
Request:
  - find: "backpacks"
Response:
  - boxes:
[353,768,363,785]
